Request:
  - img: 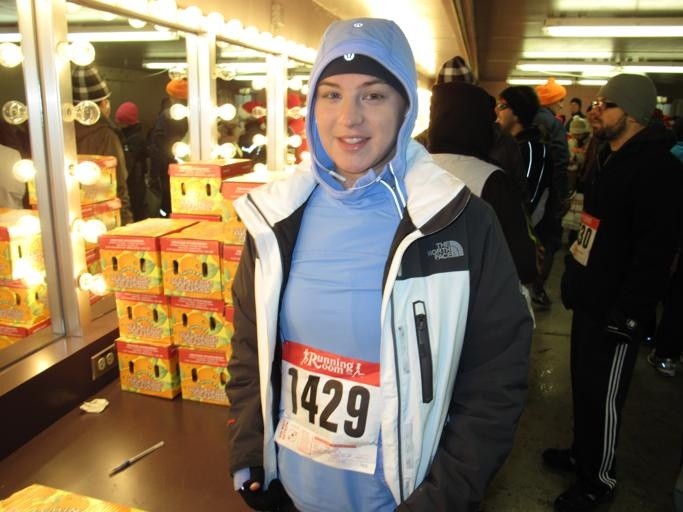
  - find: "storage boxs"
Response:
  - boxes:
[1,154,122,352]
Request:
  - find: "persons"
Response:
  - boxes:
[422,80,546,331]
[234,100,270,166]
[72,63,135,230]
[646,248,681,381]
[116,100,147,222]
[651,106,683,163]
[1,145,28,215]
[413,55,475,152]
[150,77,187,219]
[638,251,676,346]
[564,95,605,249]
[224,15,533,511]
[287,92,313,163]
[493,85,552,255]
[531,75,564,308]
[542,72,683,511]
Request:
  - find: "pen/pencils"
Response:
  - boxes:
[112,441,165,472]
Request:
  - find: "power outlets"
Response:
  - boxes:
[91,343,118,381]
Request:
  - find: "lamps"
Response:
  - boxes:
[0,0,66,372]
[505,78,609,87]
[542,0,683,38]
[516,59,683,74]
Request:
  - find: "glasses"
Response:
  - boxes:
[497,102,506,109]
[592,100,617,111]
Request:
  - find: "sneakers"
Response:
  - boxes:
[553,487,605,511]
[646,348,680,378]
[541,447,574,473]
[531,286,551,312]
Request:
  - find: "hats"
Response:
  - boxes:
[534,77,567,106]
[598,72,657,127]
[73,65,111,104]
[114,101,139,126]
[498,85,540,125]
[166,80,188,102]
[570,114,591,135]
[319,53,410,107]
[437,56,473,85]
